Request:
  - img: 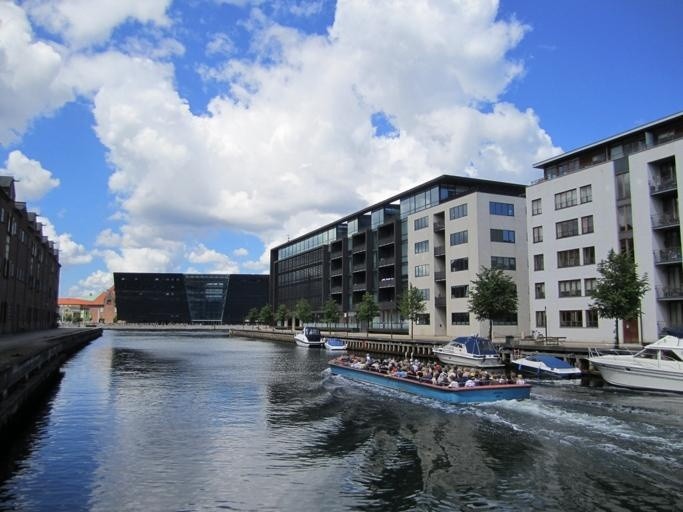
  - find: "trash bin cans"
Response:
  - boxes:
[506,335,514,344]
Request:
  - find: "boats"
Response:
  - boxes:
[328,355,533,404]
[432,336,506,368]
[293,327,348,350]
[511,353,583,388]
[587,334,682,393]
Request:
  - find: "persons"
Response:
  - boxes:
[345,352,526,389]
